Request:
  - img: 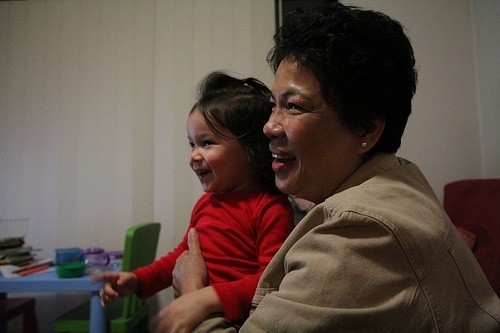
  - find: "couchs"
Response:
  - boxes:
[443,179,500,299]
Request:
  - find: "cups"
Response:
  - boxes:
[77,247,110,275]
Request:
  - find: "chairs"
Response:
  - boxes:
[51,221,160,333]
[0,291,41,333]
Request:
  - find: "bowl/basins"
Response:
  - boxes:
[54,261,85,278]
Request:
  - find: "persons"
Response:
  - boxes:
[90,70,297,333]
[173,3,500,333]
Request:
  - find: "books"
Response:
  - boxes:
[0,237,53,278]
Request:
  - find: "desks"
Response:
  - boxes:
[0,260,123,332]
